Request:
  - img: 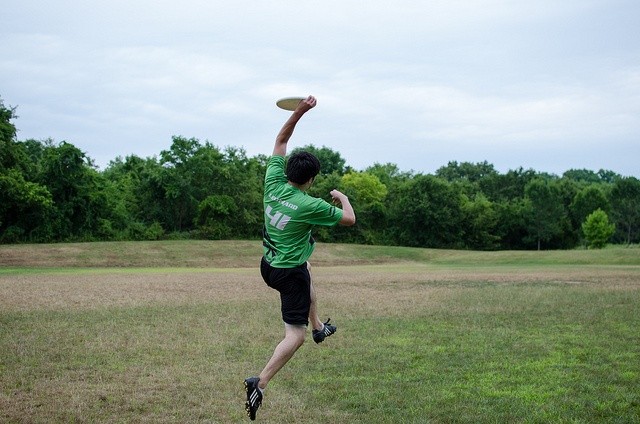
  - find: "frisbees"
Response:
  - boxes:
[276,96,306,111]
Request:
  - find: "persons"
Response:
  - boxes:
[244,95,356,420]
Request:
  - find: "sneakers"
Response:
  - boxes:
[242,377,263,421]
[312,319,337,344]
